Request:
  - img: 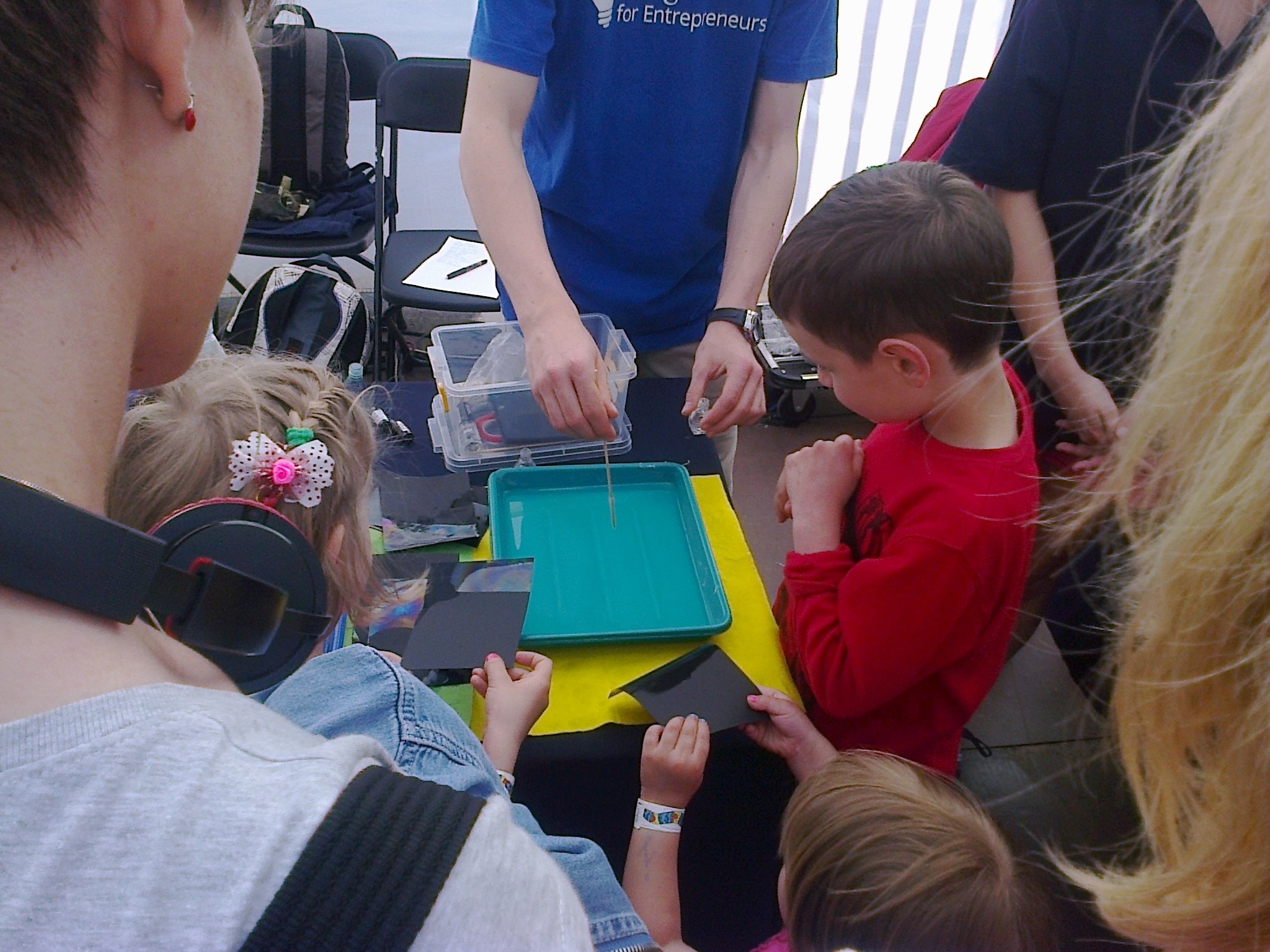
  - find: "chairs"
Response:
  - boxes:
[212,32,398,382]
[374,56,501,379]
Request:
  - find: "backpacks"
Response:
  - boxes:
[245,3,350,190]
[215,254,373,389]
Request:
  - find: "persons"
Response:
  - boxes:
[940,1,1270,716]
[0,0,588,952]
[1057,42,1270,952]
[619,687,1033,952]
[461,0,837,509]
[767,160,1039,786]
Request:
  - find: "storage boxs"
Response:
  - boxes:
[427,314,639,455]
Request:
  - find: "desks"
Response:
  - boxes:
[347,376,812,951]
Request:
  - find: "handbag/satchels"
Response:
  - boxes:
[249,182,311,221]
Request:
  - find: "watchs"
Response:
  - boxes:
[705,308,764,346]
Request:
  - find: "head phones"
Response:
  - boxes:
[1,473,335,689]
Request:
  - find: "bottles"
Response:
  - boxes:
[370,407,390,429]
[514,448,535,469]
[688,398,709,435]
[459,401,482,453]
[392,420,413,444]
[343,362,363,397]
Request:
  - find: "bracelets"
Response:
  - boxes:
[633,799,686,834]
[107,351,553,798]
[495,769,515,797]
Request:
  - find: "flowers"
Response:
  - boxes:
[257,457,305,506]
